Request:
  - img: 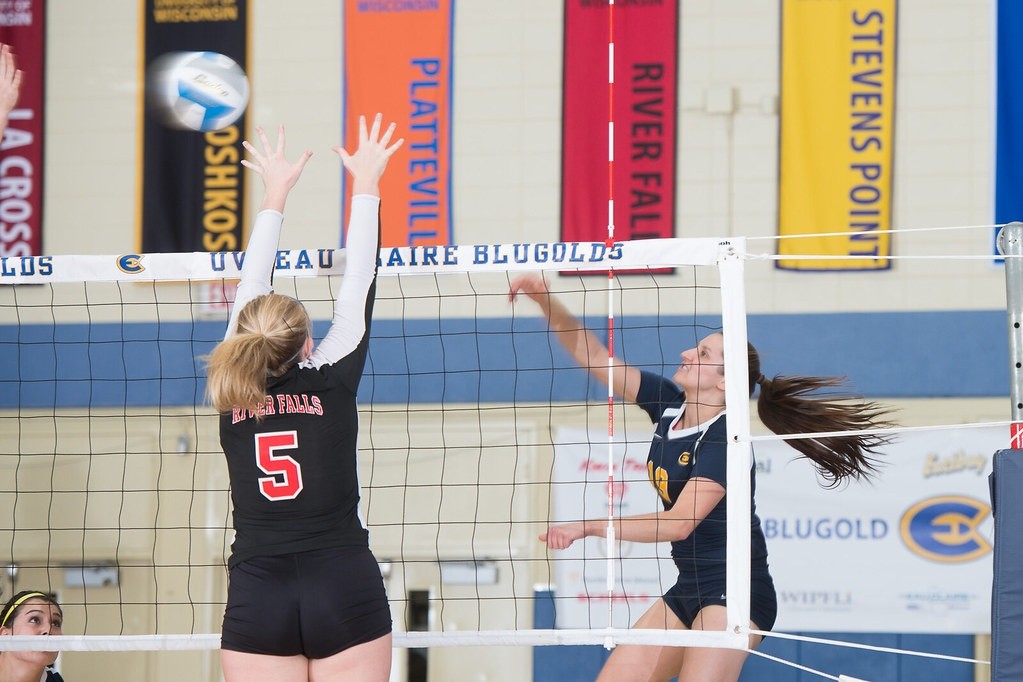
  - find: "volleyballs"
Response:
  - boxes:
[168,51,250,132]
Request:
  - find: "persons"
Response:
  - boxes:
[0,590,65,682]
[0,43,21,142]
[205,112,404,682]
[507,272,897,682]
